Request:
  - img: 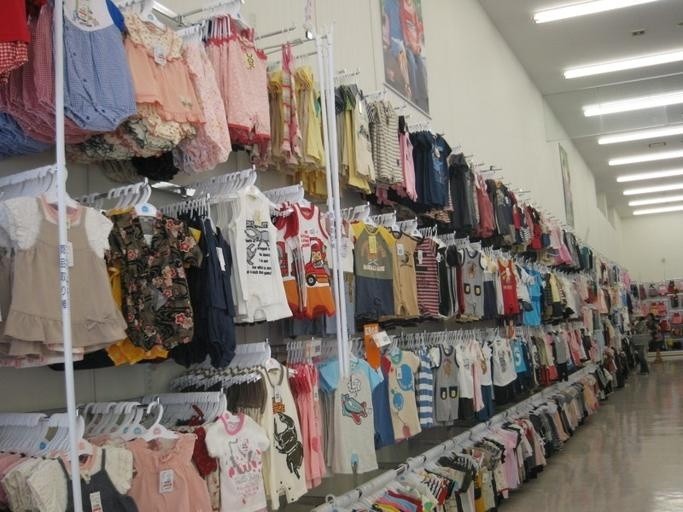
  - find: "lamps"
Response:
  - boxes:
[528,0,683,216]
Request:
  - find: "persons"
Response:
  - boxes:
[649,315,665,363]
[630,315,651,376]
